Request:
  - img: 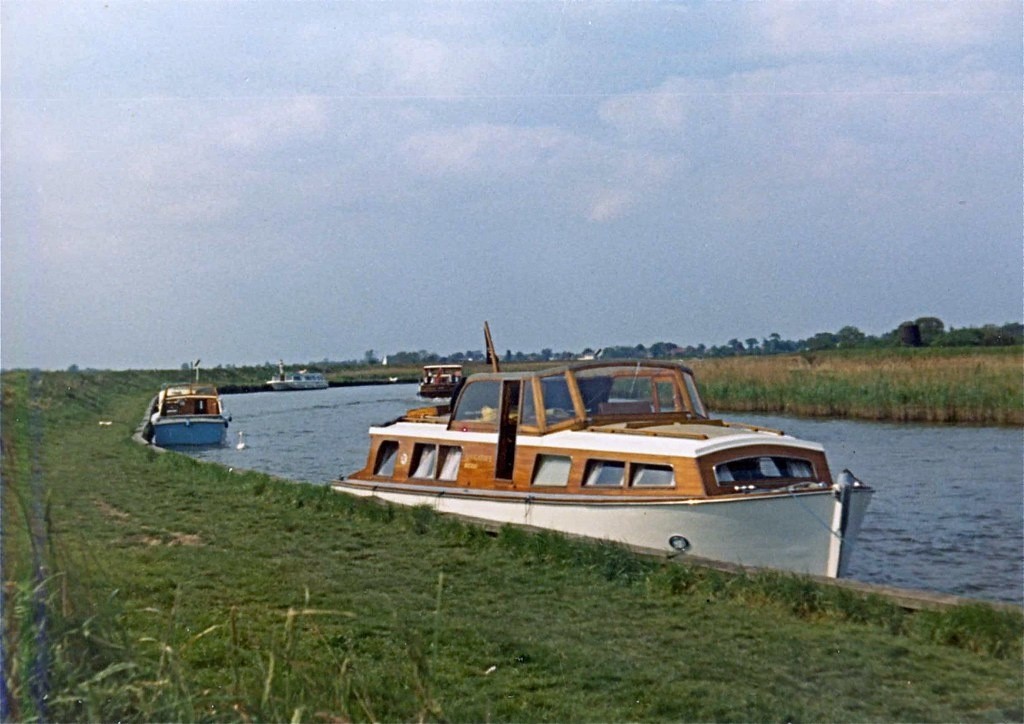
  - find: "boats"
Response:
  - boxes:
[266,359,329,391]
[415,364,464,402]
[328,319,877,582]
[149,357,234,448]
